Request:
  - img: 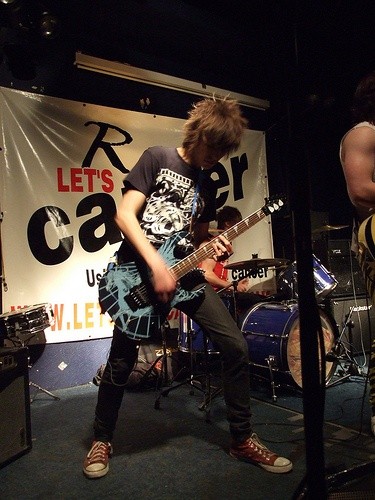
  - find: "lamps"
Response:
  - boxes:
[0,0,59,58]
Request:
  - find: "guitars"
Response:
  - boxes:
[358,215,375,262]
[98,192,289,340]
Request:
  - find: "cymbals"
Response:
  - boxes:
[311,225,351,234]
[222,258,291,270]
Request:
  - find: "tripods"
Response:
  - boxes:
[139,301,226,422]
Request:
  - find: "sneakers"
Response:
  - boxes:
[82,438,110,477]
[230,431,292,472]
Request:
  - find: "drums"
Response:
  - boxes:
[276,253,339,303]
[240,299,341,396]
[177,290,239,355]
[0,303,55,339]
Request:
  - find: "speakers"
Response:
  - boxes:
[312,238,375,298]
[0,347,33,466]
[324,294,375,358]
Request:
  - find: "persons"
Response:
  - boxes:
[82,92,292,478]
[193,206,249,292]
[339,75,375,434]
